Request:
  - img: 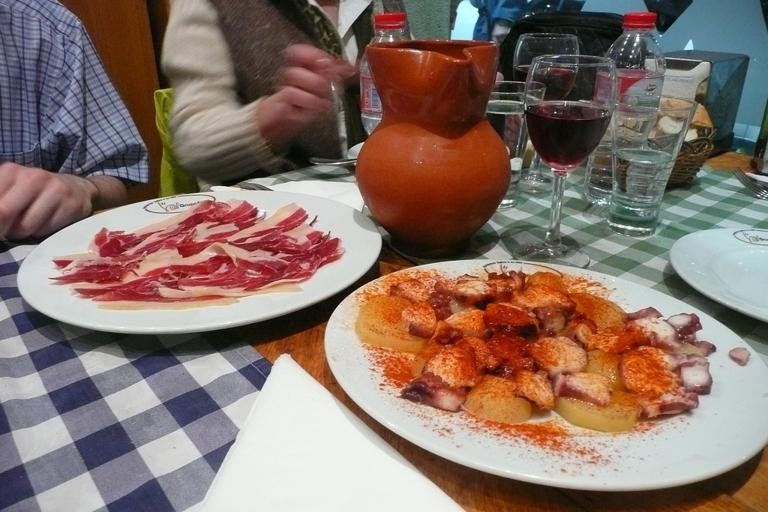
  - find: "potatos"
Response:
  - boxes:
[570,291,627,330]
[358,294,430,352]
[464,372,533,423]
[555,349,644,431]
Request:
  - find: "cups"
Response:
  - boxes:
[485,79,547,210]
[614,91,697,244]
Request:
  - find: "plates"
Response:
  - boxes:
[667,224,768,325]
[324,257,767,492]
[18,188,382,339]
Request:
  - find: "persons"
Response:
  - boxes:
[158,0,416,194]
[467,2,532,52]
[2,2,151,245]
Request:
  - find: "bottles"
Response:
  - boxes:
[583,14,666,214]
[356,11,511,259]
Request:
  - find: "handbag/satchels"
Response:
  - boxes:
[496,10,651,101]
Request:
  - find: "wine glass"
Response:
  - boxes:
[514,31,619,269]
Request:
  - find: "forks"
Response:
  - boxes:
[731,165,768,201]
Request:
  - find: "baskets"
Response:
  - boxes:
[583,123,716,191]
